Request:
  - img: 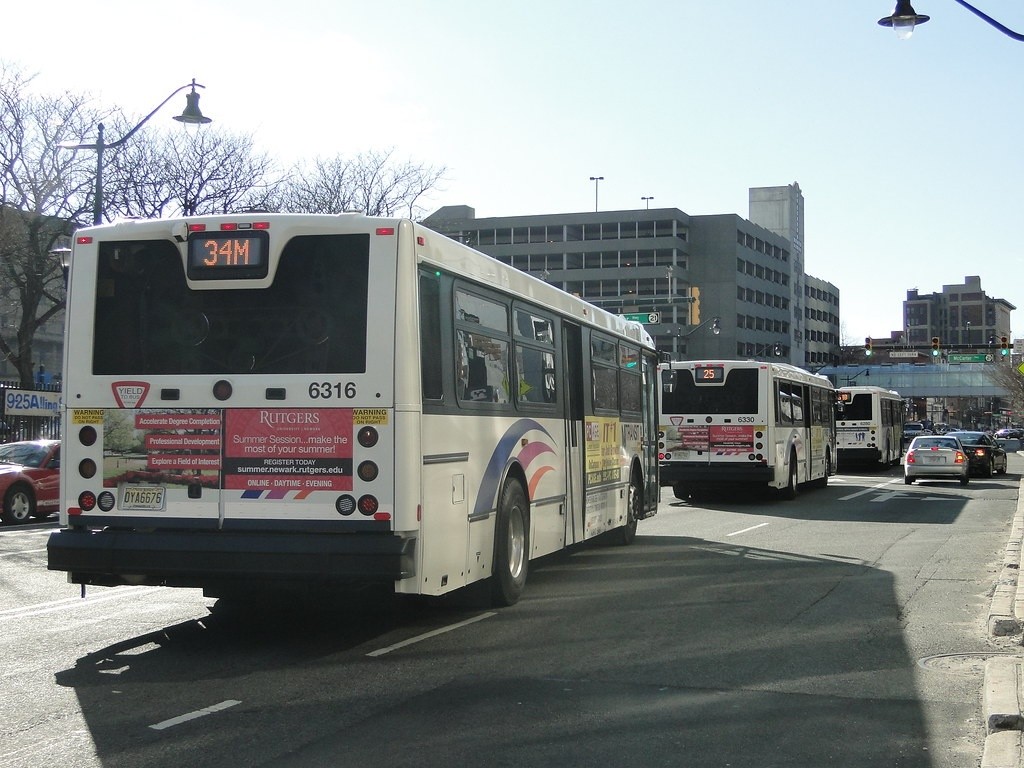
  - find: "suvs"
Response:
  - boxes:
[902,422,925,443]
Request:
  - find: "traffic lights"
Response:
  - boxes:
[1001,336,1008,355]
[932,337,940,357]
[865,337,873,357]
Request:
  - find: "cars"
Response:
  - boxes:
[0,439,62,525]
[944,432,1007,477]
[924,427,1024,439]
[903,436,971,485]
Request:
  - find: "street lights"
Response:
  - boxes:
[841,368,871,387]
[589,176,605,212]
[53,78,213,226]
[677,315,722,362]
[743,340,783,361]
[641,197,654,209]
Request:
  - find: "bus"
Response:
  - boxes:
[656,360,846,500]
[46,213,679,608]
[834,386,908,470]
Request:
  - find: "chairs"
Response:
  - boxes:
[971,440,978,444]
[944,443,954,447]
[920,444,929,447]
